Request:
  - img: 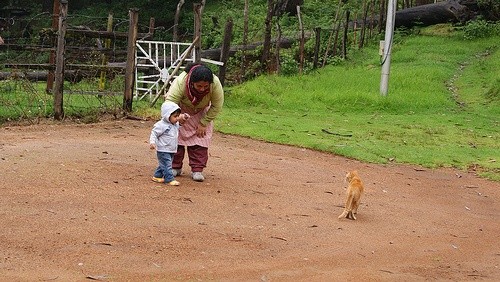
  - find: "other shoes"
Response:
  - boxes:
[167,180,180,186]
[152,176,165,183]
[171,168,181,176]
[192,172,204,181]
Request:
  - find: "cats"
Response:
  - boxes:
[338,170,364,220]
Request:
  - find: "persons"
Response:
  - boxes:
[166,62,225,182]
[149,100,189,186]
